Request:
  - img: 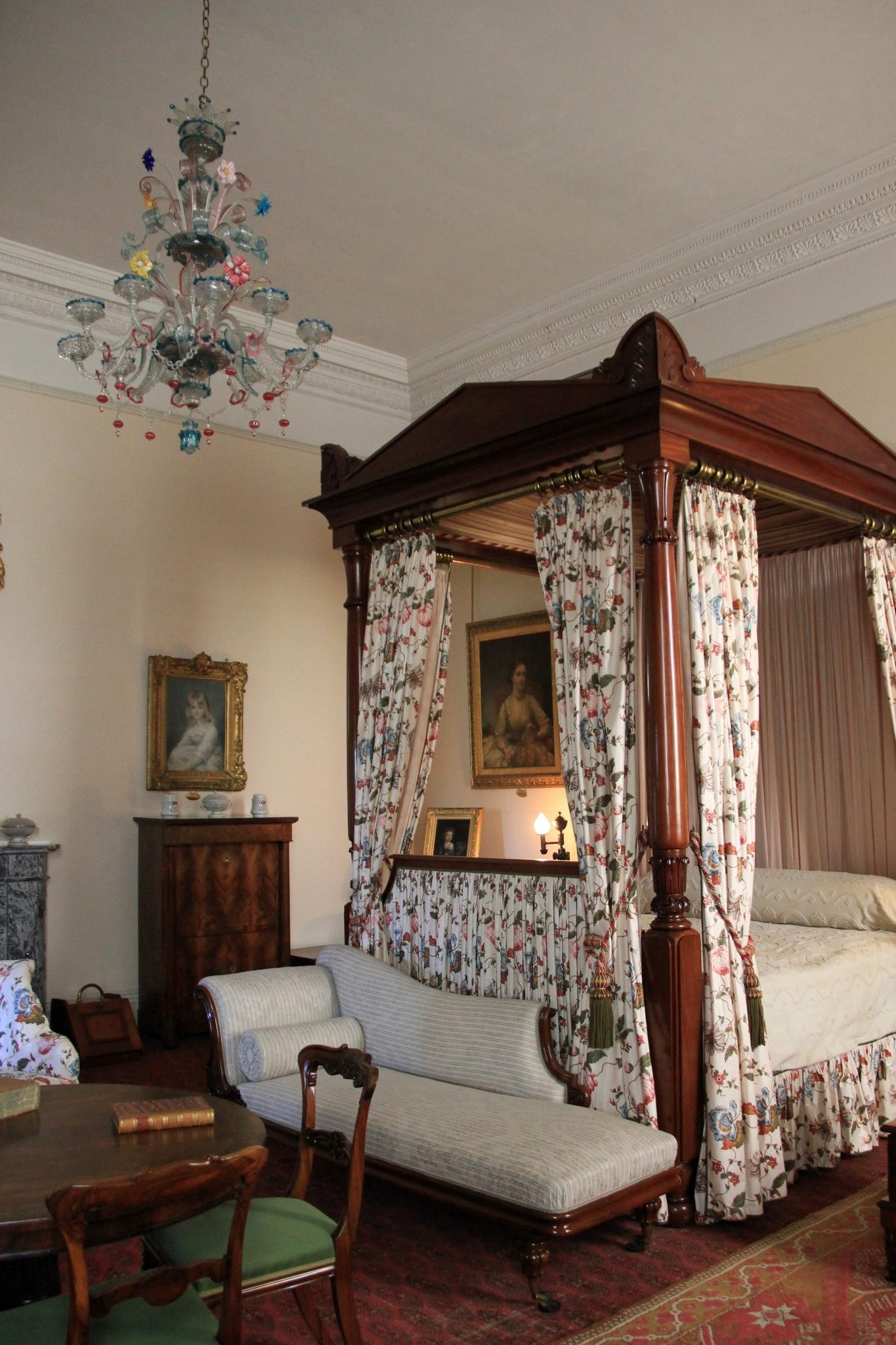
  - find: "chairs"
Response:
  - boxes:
[0,1145,268,1345]
[139,1044,378,1345]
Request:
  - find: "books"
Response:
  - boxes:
[110,1097,215,1134]
[0,1077,41,1120]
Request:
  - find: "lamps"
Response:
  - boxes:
[534,811,570,860]
[58,0,332,457]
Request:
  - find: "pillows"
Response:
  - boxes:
[749,867,896,931]
[238,1016,365,1084]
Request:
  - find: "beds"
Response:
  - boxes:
[302,310,896,1227]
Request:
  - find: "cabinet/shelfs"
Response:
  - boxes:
[132,815,299,1050]
[0,843,60,1008]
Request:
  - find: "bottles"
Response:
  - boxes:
[250,793,269,817]
[161,793,179,818]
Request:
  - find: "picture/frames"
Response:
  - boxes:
[423,807,484,858]
[147,653,246,792]
[466,610,566,790]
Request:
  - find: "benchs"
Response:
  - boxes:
[194,944,683,1312]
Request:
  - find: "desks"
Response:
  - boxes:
[0,1085,267,1311]
[291,945,327,968]
